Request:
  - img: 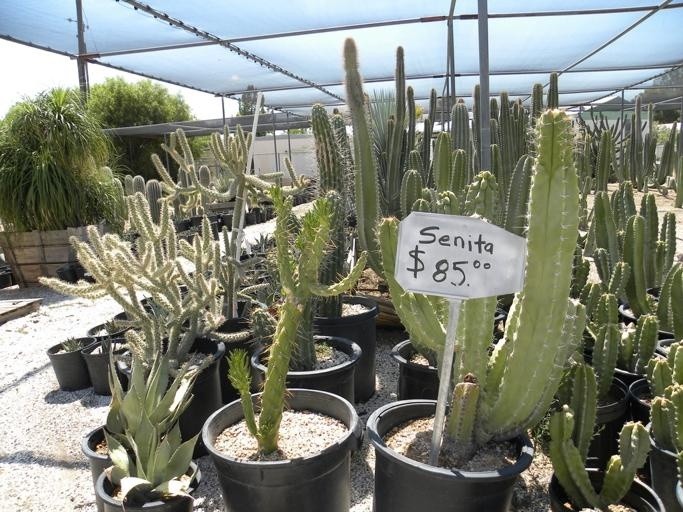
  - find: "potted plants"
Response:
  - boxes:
[0,33,683,511]
